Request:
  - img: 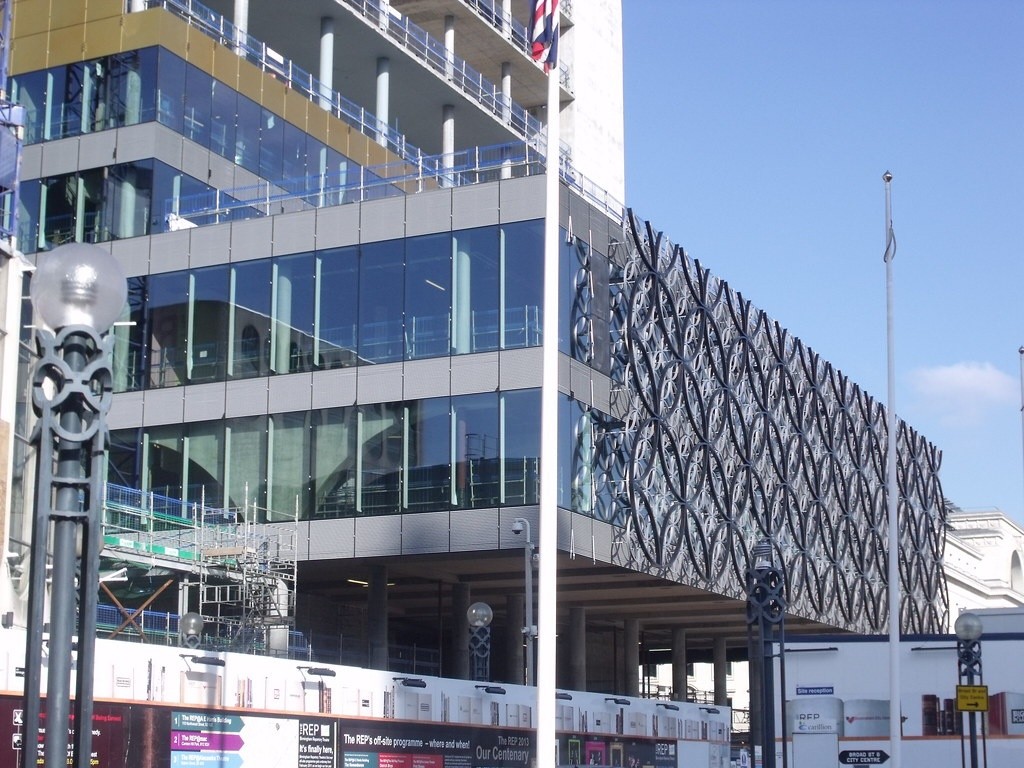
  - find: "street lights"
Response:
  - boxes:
[466,601,495,682]
[180,611,205,648]
[17,242,131,768]
[953,612,990,768]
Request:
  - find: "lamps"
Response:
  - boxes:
[475,685,507,695]
[392,677,426,688]
[605,698,630,705]
[656,703,679,710]
[698,707,719,714]
[298,665,336,677]
[180,654,226,667]
[178,612,204,635]
[40,638,79,650]
[555,692,572,700]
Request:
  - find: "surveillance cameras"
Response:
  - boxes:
[511,521,525,535]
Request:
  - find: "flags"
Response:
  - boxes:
[528,0,560,75]
